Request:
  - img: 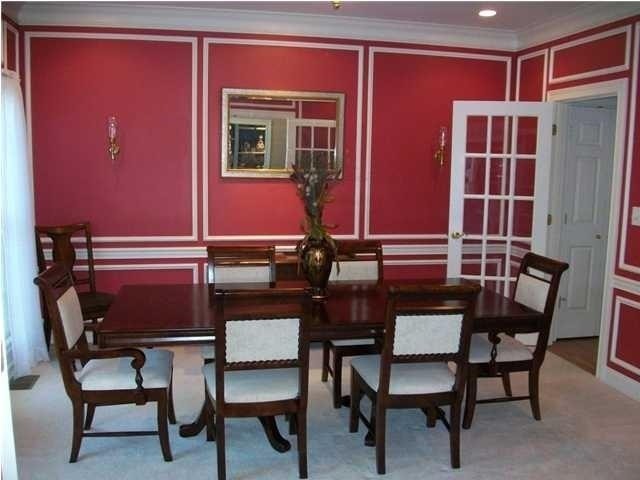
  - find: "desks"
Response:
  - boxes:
[96,278,543,454]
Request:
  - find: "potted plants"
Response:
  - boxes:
[287,159,340,300]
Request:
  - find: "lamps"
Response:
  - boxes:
[435,126,448,166]
[104,116,120,160]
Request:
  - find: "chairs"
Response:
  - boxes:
[33,264,177,463]
[33,221,115,351]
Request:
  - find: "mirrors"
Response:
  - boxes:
[220,88,345,180]
